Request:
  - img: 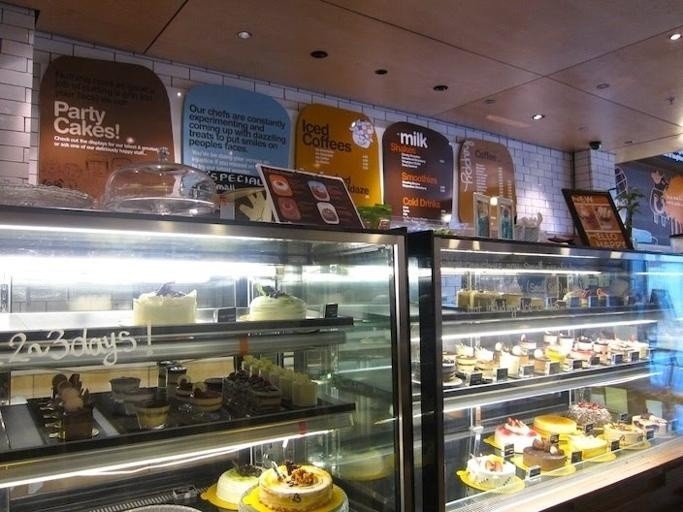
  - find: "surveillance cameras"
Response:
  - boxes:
[590,141,601,150]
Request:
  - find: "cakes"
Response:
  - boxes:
[248,285,307,321]
[132,284,197,327]
[465,399,670,488]
[216,464,268,505]
[258,459,333,512]
[455,288,601,312]
[414,332,651,387]
[52,353,319,442]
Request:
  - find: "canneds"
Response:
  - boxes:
[605,295,618,306]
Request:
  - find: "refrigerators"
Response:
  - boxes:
[0,205,415,512]
[417,230,681,511]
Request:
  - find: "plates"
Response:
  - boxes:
[105,197,219,218]
[409,374,463,387]
[3,181,96,206]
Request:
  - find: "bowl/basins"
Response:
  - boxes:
[110,376,224,431]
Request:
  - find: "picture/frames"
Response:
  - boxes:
[561,187,635,250]
[255,163,366,228]
[473,191,514,240]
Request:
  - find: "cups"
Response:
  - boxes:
[525,226,540,243]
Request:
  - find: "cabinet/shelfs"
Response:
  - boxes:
[0,205,682,512]
